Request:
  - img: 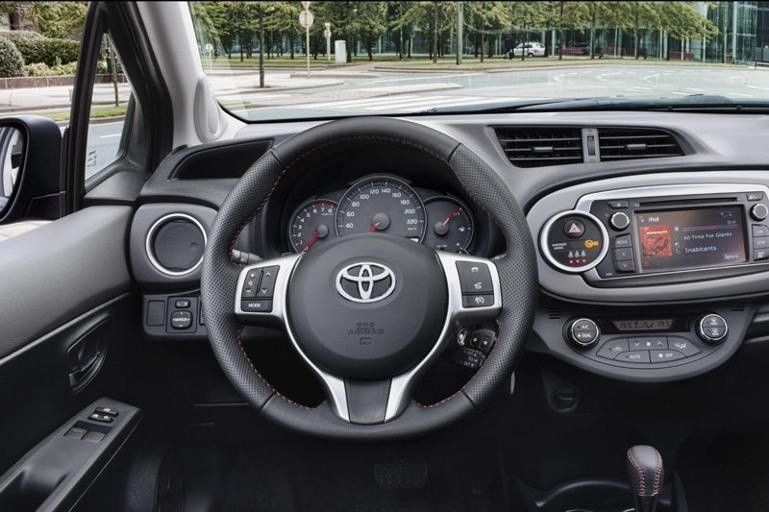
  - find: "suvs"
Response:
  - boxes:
[507,40,545,57]
[559,42,591,56]
